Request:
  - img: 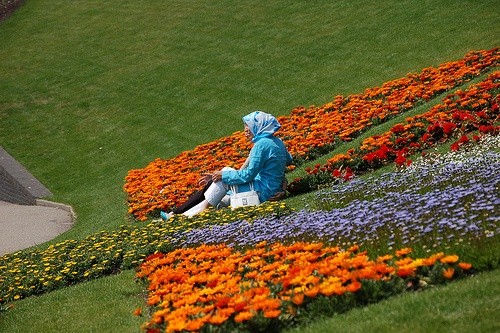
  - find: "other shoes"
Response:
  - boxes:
[160,211,167,221]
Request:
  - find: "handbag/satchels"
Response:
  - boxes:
[230,182,260,211]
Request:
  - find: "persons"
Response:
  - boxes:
[159,145,289,222]
[182,111,294,217]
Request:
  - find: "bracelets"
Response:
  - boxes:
[282,189,287,197]
